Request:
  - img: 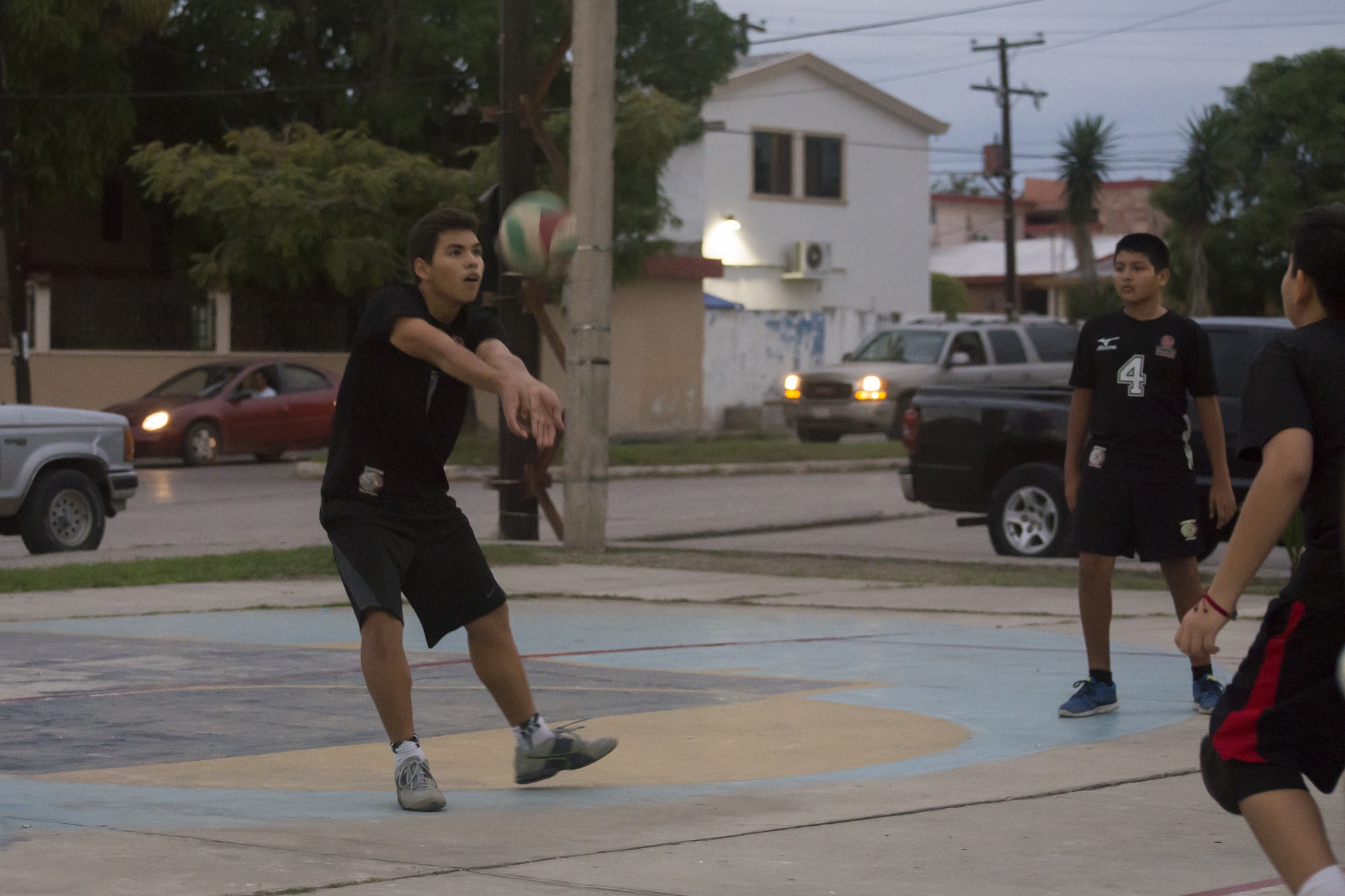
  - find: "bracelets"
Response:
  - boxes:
[1194,588,1236,622]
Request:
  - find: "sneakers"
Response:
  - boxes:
[1192,668,1223,714]
[394,754,447,812]
[1059,677,1120,717]
[514,717,618,784]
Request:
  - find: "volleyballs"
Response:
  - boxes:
[497,189,579,281]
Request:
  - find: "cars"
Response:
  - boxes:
[0,402,139,555]
[108,356,344,467]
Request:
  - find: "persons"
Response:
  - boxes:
[1056,233,1239,716]
[250,372,278,399]
[321,210,620,810]
[1175,208,1345,896]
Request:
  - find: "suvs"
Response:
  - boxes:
[779,321,1087,442]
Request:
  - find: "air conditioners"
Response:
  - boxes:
[794,241,832,276]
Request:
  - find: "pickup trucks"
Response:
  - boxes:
[897,315,1310,565]
